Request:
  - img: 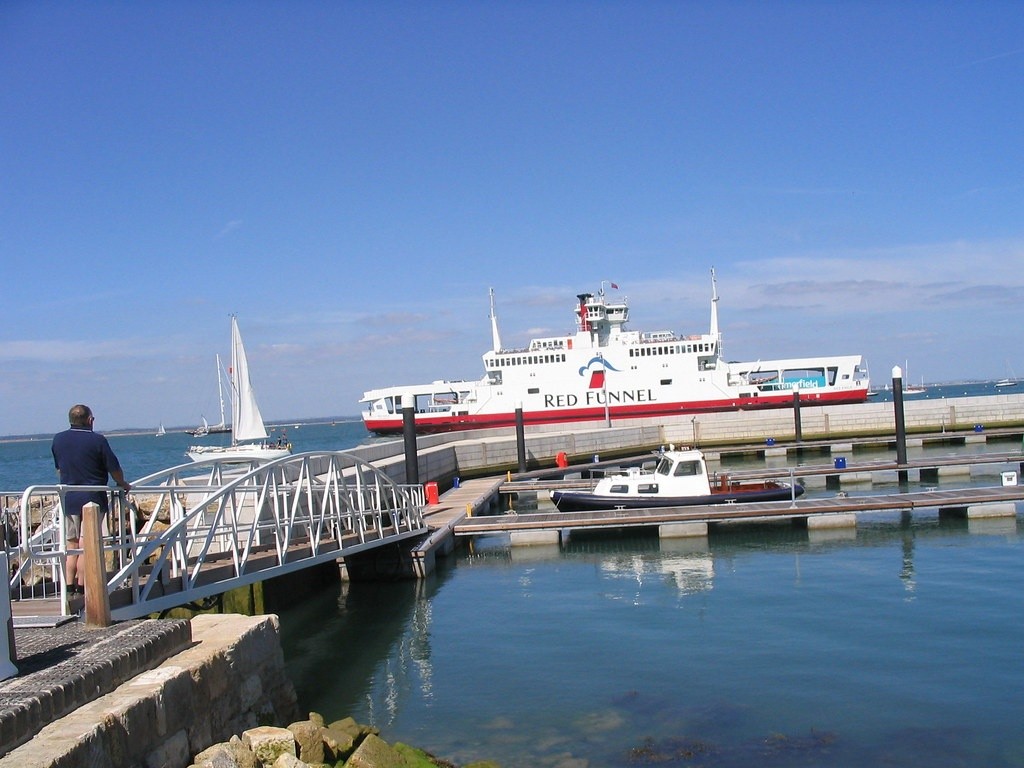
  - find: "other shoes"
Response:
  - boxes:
[73,593,81,600]
[66,592,73,602]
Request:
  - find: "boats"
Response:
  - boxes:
[995,379,1017,387]
[357,264,871,434]
[548,443,806,512]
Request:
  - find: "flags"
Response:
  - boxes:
[612,283,618,288]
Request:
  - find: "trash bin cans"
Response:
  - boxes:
[558,452,567,467]
[426,481,439,504]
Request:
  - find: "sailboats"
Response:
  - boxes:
[187,315,294,463]
[864,359,879,396]
[155,422,166,436]
[901,359,928,394]
[184,351,233,435]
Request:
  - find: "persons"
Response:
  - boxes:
[51,405,132,600]
[276,433,287,447]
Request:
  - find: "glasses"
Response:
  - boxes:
[89,417,95,421]
